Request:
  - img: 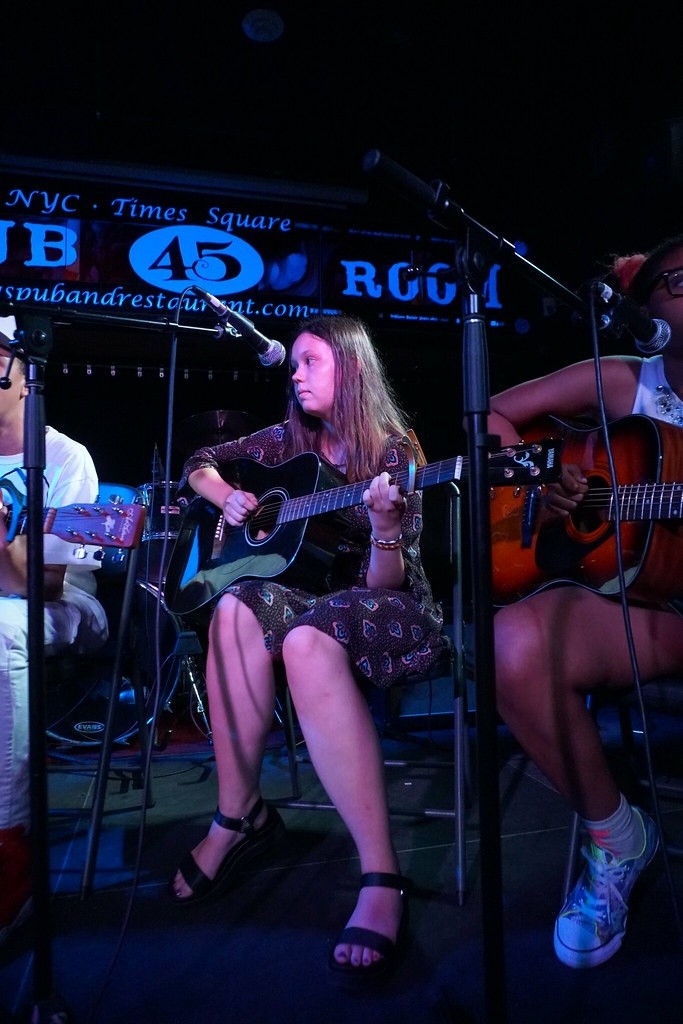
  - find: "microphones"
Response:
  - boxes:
[592,281,672,354]
[189,285,286,369]
[116,686,150,706]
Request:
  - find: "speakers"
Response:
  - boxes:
[395,606,477,735]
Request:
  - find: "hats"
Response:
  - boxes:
[0,314,24,353]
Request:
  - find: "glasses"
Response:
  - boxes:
[639,266,683,306]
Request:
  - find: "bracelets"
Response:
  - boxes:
[369,531,404,549]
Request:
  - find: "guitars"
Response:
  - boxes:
[489,412,683,610]
[0,494,149,563]
[163,433,564,619]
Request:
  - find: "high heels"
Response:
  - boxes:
[166,794,285,911]
[327,872,411,974]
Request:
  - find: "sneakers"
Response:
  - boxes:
[551,804,660,970]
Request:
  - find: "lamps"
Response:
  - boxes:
[269,251,308,299]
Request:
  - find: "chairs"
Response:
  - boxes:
[40,480,502,907]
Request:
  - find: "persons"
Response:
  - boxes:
[486,248,683,968]
[0,345,109,939]
[171,316,444,975]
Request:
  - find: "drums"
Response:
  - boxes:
[44,480,189,746]
